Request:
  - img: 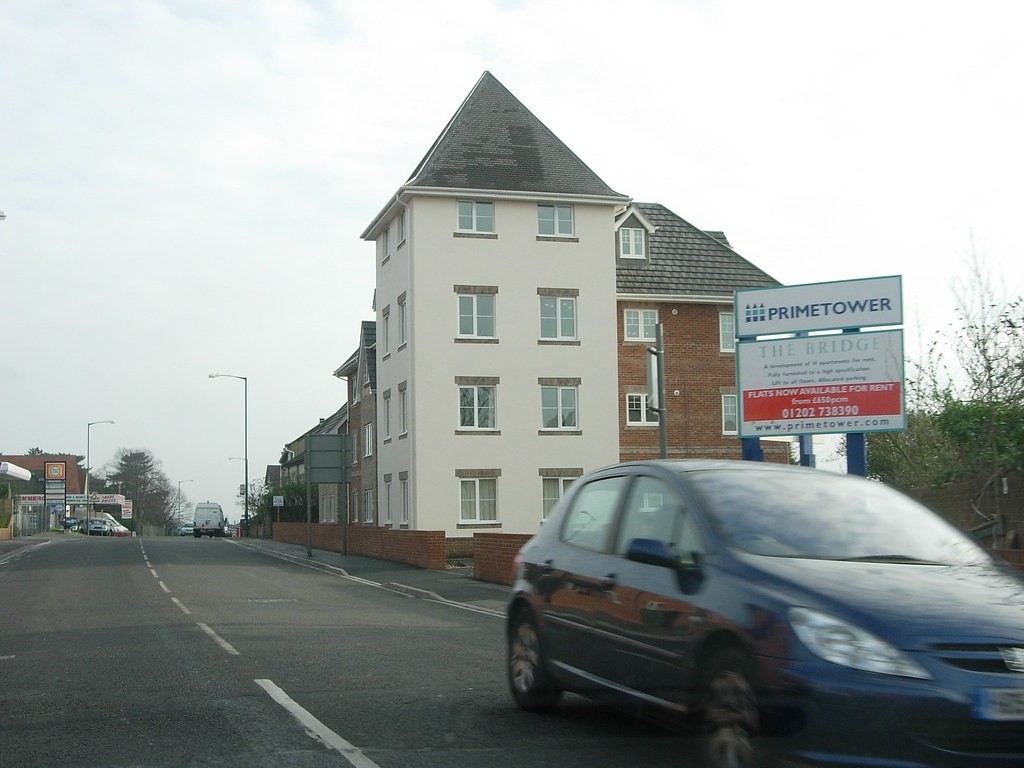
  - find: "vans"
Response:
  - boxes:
[72,511,129,535]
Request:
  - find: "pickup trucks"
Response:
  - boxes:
[88,518,113,536]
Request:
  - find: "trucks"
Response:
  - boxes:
[193,501,227,538]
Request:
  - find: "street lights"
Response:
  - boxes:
[229,458,248,537]
[86,421,115,535]
[179,480,194,524]
[209,374,248,537]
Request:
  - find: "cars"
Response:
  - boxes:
[505,458,1024,768]
[181,522,194,536]
